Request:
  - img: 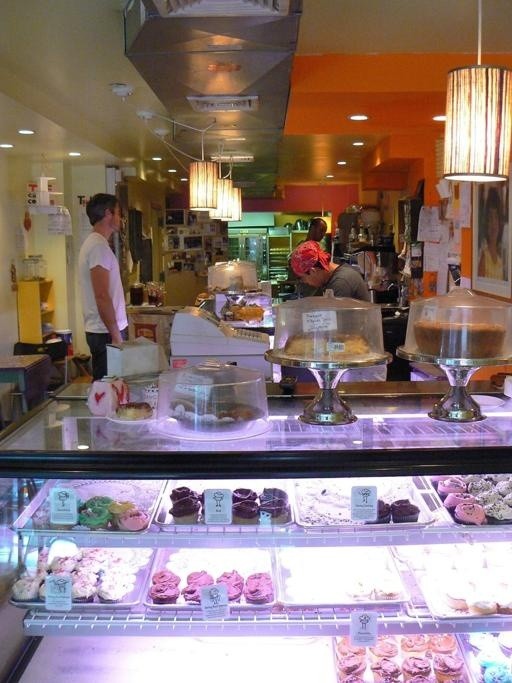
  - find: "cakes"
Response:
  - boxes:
[337,657,366,677]
[483,501,512,524]
[181,584,201,604]
[376,677,396,683]
[339,638,368,657]
[259,488,288,505]
[284,334,366,357]
[169,486,199,505]
[233,488,257,502]
[217,581,241,603]
[232,500,258,524]
[443,491,474,508]
[341,674,366,683]
[455,502,485,525]
[259,498,291,524]
[503,493,512,507]
[117,511,147,530]
[367,499,390,523]
[462,474,479,482]
[169,495,200,524]
[243,584,274,605]
[215,570,242,592]
[475,490,501,505]
[109,500,134,513]
[467,479,491,492]
[426,635,454,654]
[85,497,109,509]
[404,676,432,683]
[369,658,401,682]
[14,546,134,604]
[152,571,180,586]
[149,583,180,604]
[245,572,272,586]
[415,320,506,359]
[401,636,428,656]
[495,481,512,496]
[368,640,394,660]
[392,499,419,523]
[433,656,464,682]
[492,477,509,485]
[187,570,214,586]
[79,508,110,529]
[402,657,431,681]
[437,477,466,497]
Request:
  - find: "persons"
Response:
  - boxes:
[290,241,370,302]
[478,187,507,280]
[79,193,129,382]
[293,218,326,298]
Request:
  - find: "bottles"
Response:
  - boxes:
[358,223,367,241]
[367,225,376,241]
[21,254,47,281]
[348,223,357,239]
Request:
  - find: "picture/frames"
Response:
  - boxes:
[470,176,512,303]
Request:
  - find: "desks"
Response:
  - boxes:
[0,355,50,413]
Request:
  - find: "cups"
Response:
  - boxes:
[55,329,72,344]
[147,287,158,305]
[129,283,145,305]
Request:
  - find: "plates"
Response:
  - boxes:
[106,409,156,425]
[170,402,264,433]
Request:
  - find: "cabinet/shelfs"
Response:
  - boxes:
[164,208,227,270]
[18,278,56,344]
[0,379,512,683]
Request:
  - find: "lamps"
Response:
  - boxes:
[209,140,233,220]
[221,154,242,222]
[188,122,218,211]
[442,0,512,183]
